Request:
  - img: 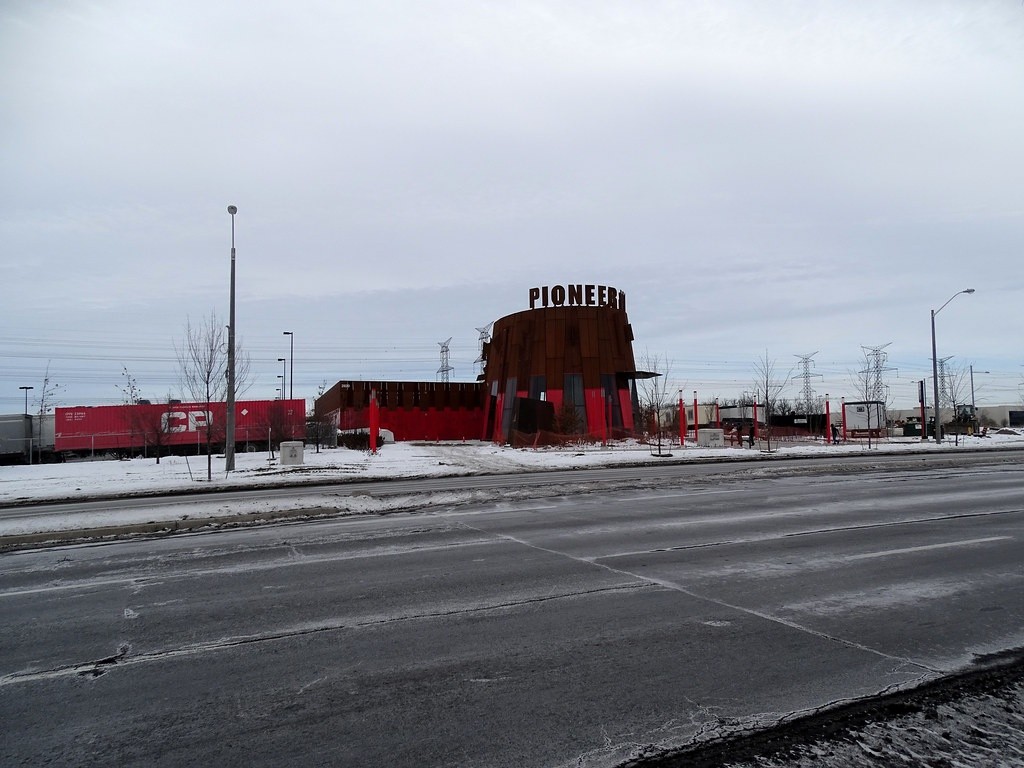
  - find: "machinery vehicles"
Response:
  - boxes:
[943,403,979,436]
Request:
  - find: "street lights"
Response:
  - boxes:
[929,288,976,445]
[276,388,281,400]
[18,386,34,465]
[970,365,990,414]
[278,358,285,401]
[277,375,283,400]
[224,203,238,473]
[283,331,293,400]
